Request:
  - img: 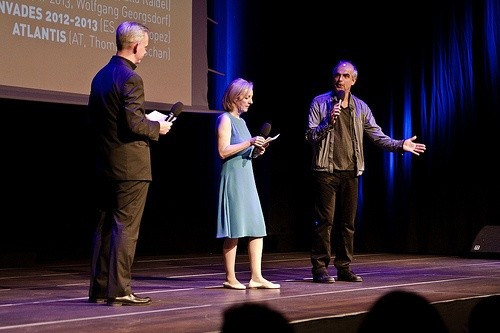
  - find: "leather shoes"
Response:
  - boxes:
[107,292,151,307]
[89,296,107,303]
[223,280,244,289]
[249,278,280,289]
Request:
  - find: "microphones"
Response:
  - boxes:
[251,123,271,158]
[334,90,345,121]
[165,101,185,122]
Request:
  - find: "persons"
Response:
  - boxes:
[217,78,280,290]
[360,290,447,333]
[88,21,173,308]
[305,60,426,283]
[220,302,295,333]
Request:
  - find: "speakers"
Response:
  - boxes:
[471,225,500,259]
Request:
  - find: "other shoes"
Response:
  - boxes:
[337,273,362,281]
[313,274,335,283]
